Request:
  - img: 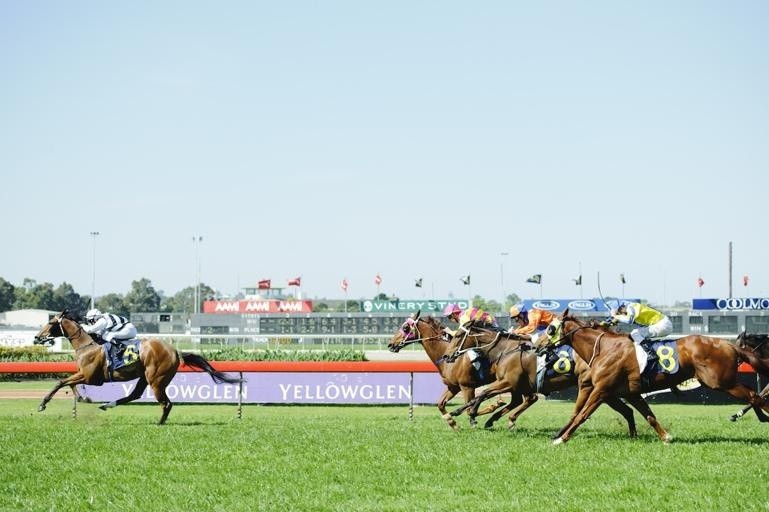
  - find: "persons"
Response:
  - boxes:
[610,300,673,343]
[508,303,558,335]
[80,309,137,370]
[443,303,497,337]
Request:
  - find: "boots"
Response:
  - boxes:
[110,338,126,357]
[640,339,660,361]
[548,350,559,365]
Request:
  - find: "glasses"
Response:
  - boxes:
[447,315,453,319]
[616,311,622,314]
[514,316,519,321]
[88,319,94,323]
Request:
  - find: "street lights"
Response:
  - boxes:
[89,232,101,310]
[192,235,203,314]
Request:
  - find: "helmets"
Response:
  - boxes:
[85,309,100,319]
[609,302,623,314]
[443,306,461,317]
[510,304,527,319]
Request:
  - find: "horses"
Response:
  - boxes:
[730,330,769,421]
[443,319,674,446]
[388,310,538,435]
[33,306,248,425]
[535,307,769,447]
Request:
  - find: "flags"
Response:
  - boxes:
[527,275,541,283]
[618,274,625,284]
[289,277,300,286]
[342,279,347,290]
[743,274,749,286]
[699,279,704,287]
[576,275,581,285]
[259,280,270,288]
[376,276,381,284]
[460,275,470,284]
[416,278,422,287]
